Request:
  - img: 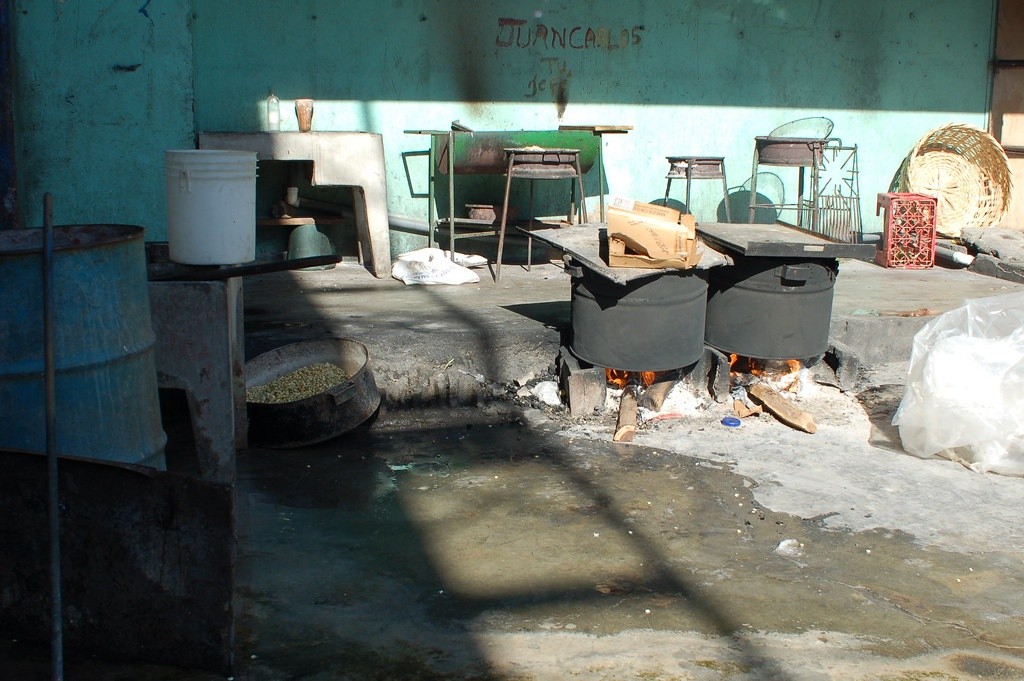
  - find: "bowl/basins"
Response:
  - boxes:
[503,148,576,169]
[755,136,829,167]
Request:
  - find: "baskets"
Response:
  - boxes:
[899,122,1013,238]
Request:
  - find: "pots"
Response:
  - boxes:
[245,336,381,446]
[704,246,840,360]
[562,254,709,371]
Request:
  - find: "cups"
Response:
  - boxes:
[296,99,313,133]
[287,187,298,205]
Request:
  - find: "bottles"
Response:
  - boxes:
[267,88,280,133]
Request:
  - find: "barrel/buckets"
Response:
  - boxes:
[163,149,259,265]
[0,223,168,473]
